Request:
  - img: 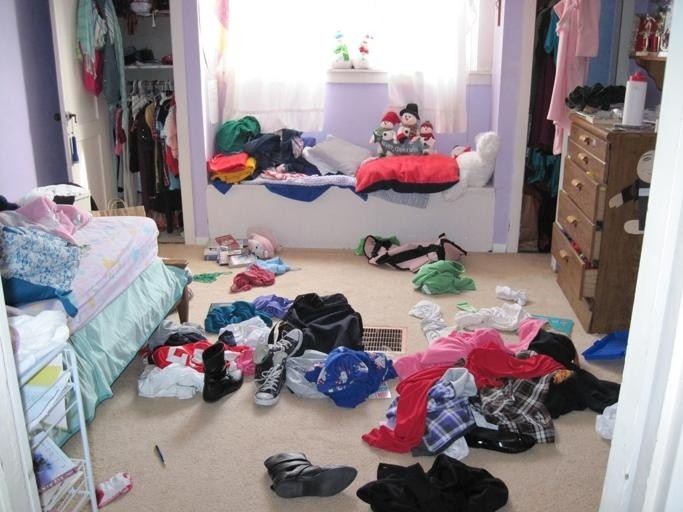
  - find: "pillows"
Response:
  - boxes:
[309,134,371,177]
[354,153,459,195]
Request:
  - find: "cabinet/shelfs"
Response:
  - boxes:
[124,10,173,69]
[550,113,656,335]
[49,1,197,247]
[18,343,99,512]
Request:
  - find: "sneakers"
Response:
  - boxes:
[252,328,303,406]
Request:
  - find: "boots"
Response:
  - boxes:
[264,453,357,498]
[202,343,243,403]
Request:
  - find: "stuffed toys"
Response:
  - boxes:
[349,35,378,70]
[441,131,500,203]
[245,227,282,259]
[368,112,402,159]
[330,30,351,69]
[395,103,421,155]
[408,121,436,155]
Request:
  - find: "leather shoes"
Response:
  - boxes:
[464,426,536,454]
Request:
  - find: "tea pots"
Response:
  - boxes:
[632,12,660,57]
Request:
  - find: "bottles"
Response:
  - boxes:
[622,71,648,127]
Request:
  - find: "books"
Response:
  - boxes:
[20,364,71,431]
[28,430,79,495]
[213,233,242,255]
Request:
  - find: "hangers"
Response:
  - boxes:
[128,80,172,105]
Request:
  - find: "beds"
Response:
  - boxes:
[8,216,189,462]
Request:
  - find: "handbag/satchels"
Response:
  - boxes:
[92,199,146,217]
